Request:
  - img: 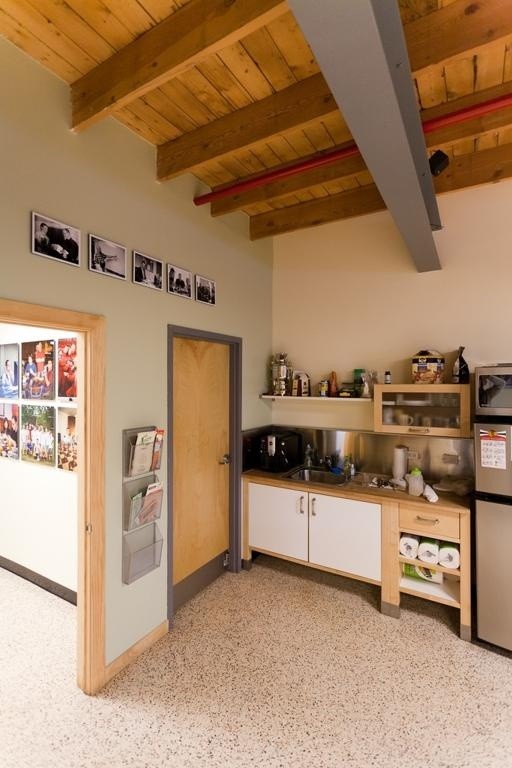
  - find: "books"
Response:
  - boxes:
[128,491,143,529]
[150,427,165,470]
[131,431,158,475]
[143,481,164,521]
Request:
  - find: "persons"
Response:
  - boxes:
[169,267,215,304]
[137,260,147,281]
[57,228,78,262]
[0,341,78,472]
[479,374,505,407]
[95,244,117,272]
[36,222,50,251]
[145,264,155,285]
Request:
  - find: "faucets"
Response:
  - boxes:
[323,456,332,469]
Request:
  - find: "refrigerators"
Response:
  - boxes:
[472,420,511,655]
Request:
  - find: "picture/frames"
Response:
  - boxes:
[166,263,193,299]
[132,249,164,291]
[195,274,217,307]
[30,210,81,266]
[88,232,128,281]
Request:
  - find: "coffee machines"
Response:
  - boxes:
[255,432,302,472]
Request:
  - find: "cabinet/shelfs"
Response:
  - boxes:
[241,459,388,619]
[372,383,470,440]
[388,473,475,643]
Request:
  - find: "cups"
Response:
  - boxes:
[385,409,460,427]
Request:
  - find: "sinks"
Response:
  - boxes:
[287,469,345,485]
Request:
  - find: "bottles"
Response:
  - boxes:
[318,368,366,397]
[344,455,355,476]
[409,467,423,496]
[384,370,392,384]
